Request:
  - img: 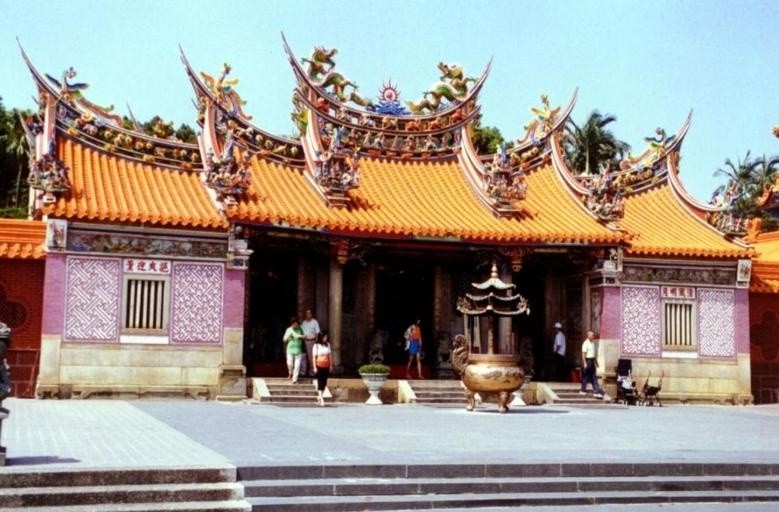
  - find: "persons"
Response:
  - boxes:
[579,331,603,398]
[552,322,566,363]
[403,319,424,379]
[282,309,332,406]
[0,358,11,383]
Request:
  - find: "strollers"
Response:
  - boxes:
[615,360,664,406]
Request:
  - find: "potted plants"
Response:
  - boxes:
[357,362,392,406]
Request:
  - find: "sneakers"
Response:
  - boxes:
[579,391,602,398]
